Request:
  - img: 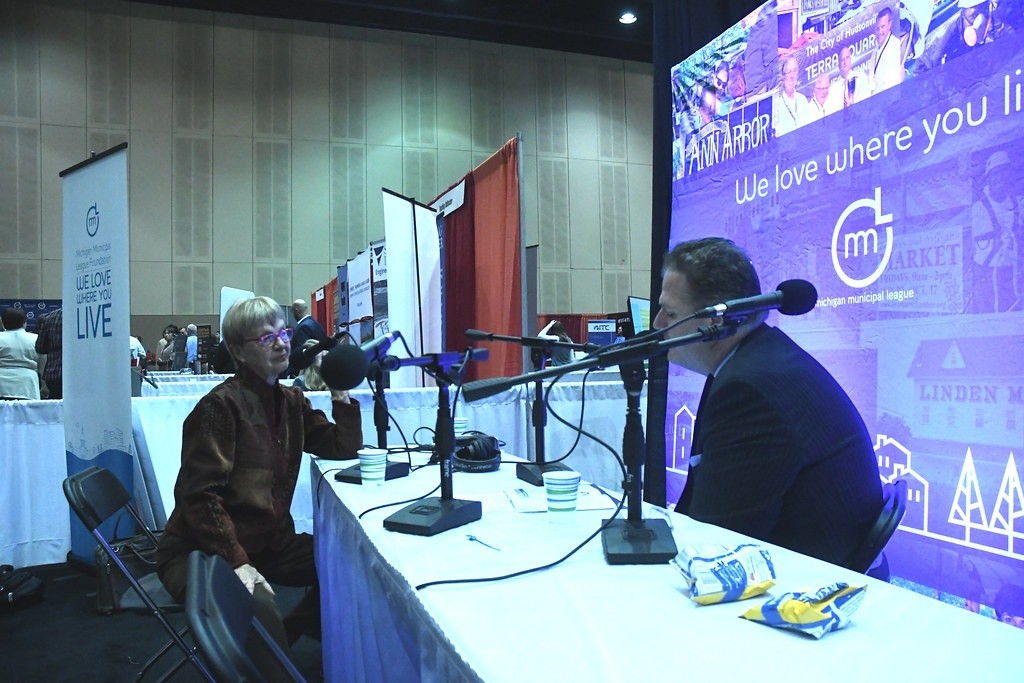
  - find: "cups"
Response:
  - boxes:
[453,418,468,437]
[542,471,581,515]
[357,449,388,489]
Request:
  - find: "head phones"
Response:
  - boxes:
[452,437,501,472]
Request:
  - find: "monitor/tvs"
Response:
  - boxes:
[627,296,652,336]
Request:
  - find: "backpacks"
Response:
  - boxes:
[0,564,42,612]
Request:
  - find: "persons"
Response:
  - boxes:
[2,298,334,399]
[156,297,364,683]
[653,237,885,573]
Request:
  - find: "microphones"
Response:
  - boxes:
[289,331,346,371]
[320,331,400,391]
[589,330,665,372]
[695,280,818,318]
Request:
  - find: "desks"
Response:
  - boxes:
[309,445,1024,683]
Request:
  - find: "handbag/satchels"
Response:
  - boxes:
[95,510,184,617]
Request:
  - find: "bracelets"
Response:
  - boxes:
[332,393,349,402]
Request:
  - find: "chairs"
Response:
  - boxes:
[62,465,218,683]
[844,477,908,576]
[185,551,308,683]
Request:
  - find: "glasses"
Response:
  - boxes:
[243,328,293,346]
